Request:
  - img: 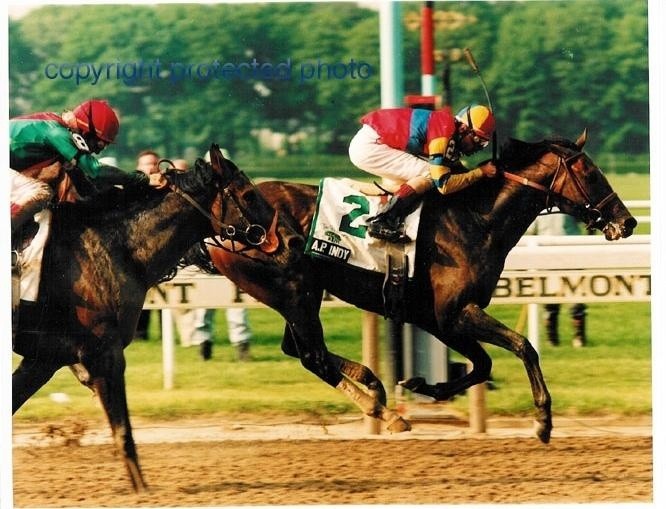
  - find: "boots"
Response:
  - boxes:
[368,197,413,244]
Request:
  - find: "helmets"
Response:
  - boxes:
[63,98,118,144]
[456,103,495,142]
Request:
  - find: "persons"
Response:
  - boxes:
[528,207,588,349]
[189,147,253,363]
[133,151,189,340]
[10,99,147,235]
[346,102,498,244]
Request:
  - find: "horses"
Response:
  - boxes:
[177,128,639,446]
[9,140,308,494]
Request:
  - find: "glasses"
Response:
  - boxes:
[467,123,489,149]
